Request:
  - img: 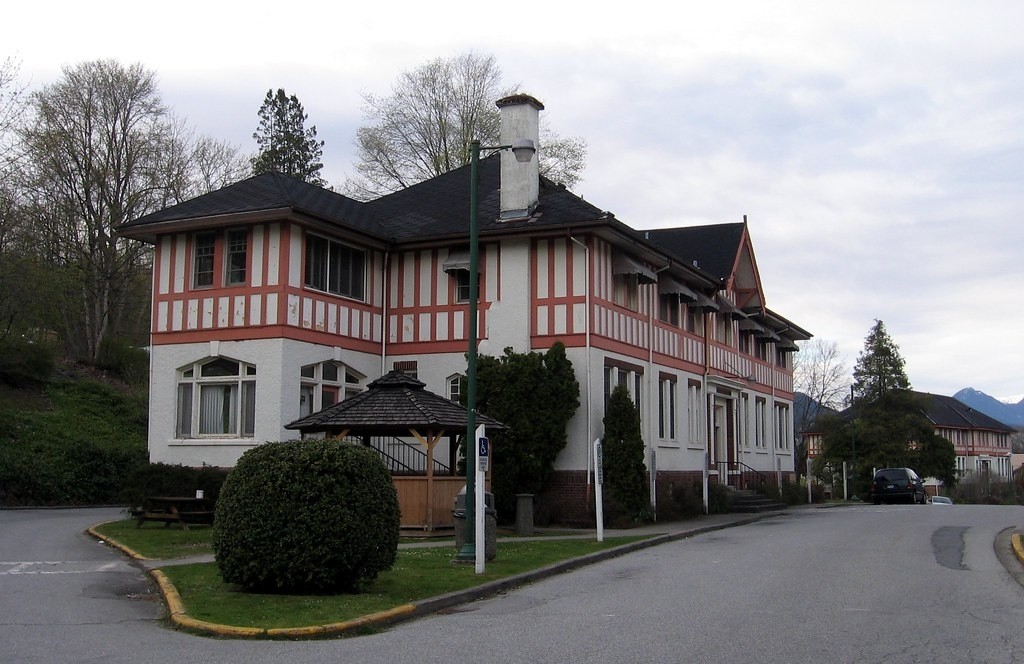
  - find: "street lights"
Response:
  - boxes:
[452,135,538,566]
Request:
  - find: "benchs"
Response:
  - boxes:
[132,497,214,531]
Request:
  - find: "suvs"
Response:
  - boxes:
[871,467,928,504]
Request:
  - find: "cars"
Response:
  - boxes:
[927,496,956,506]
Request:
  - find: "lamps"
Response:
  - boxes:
[725,375,756,383]
